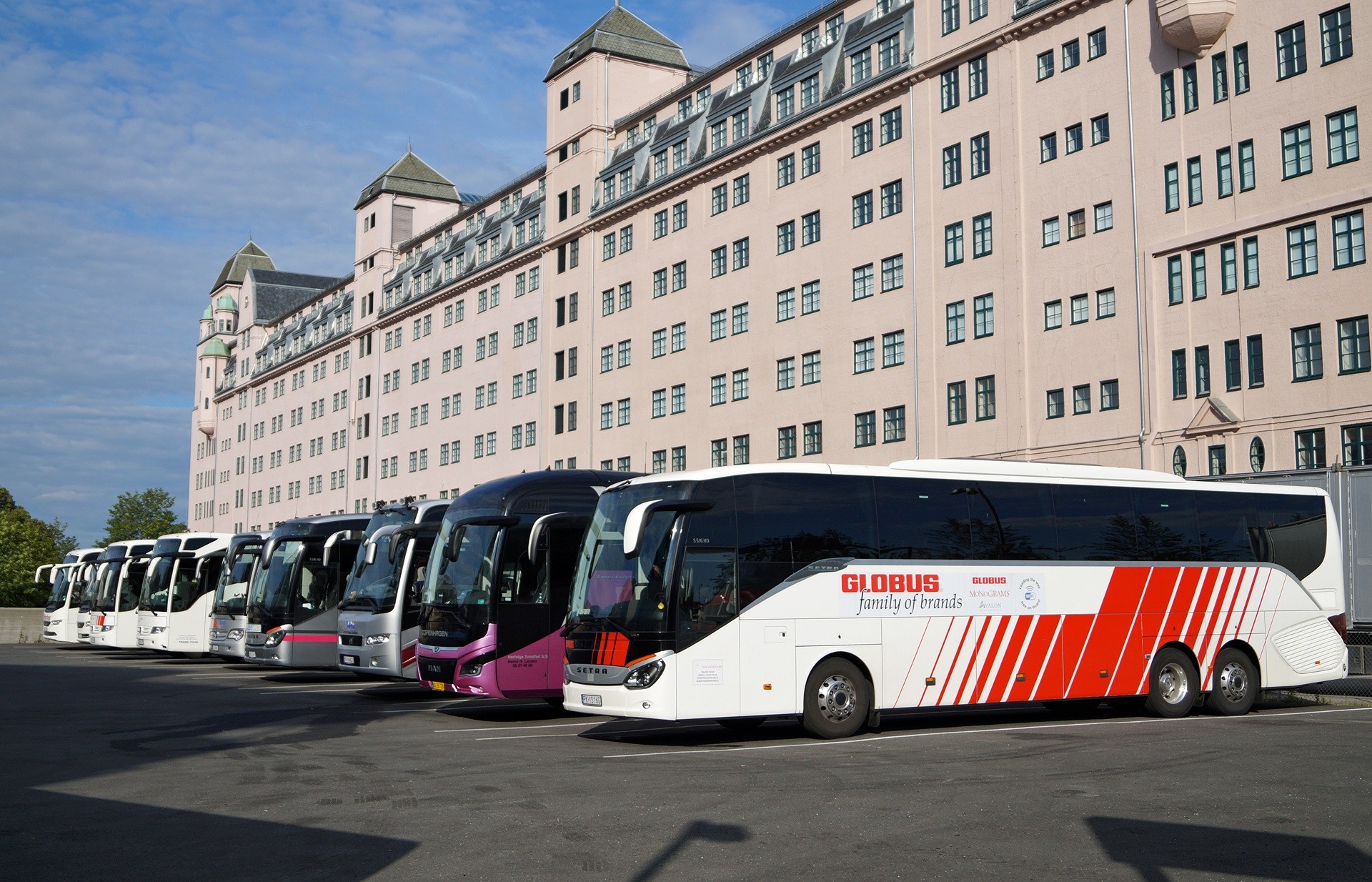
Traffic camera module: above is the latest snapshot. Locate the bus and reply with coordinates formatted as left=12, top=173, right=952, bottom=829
left=386, top=469, right=654, bottom=707
left=195, top=530, right=283, bottom=667
left=228, top=512, right=375, bottom=673
left=322, top=498, right=473, bottom=680
left=123, top=532, right=237, bottom=658
left=528, top=458, right=1350, bottom=742
left=34, top=538, right=158, bottom=650
